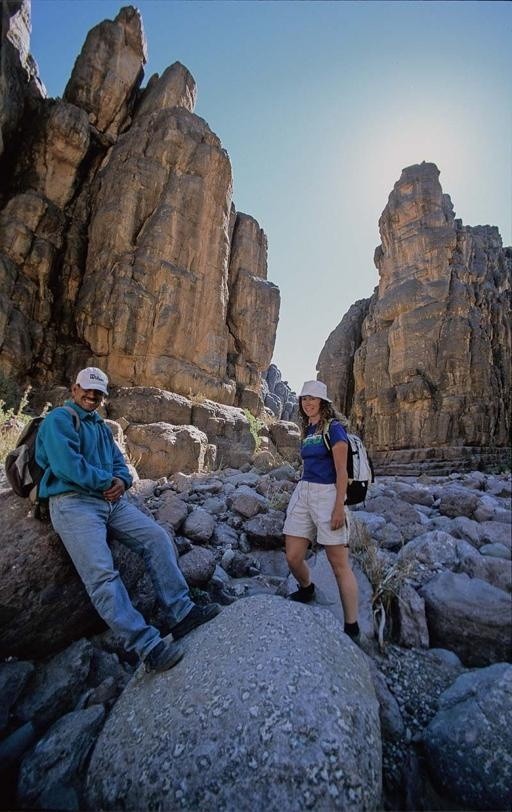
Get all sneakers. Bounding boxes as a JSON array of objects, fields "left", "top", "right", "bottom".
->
[
  {"left": 144, "top": 640, "right": 183, "bottom": 674},
  {"left": 287, "top": 584, "right": 316, "bottom": 602},
  {"left": 344, "top": 628, "right": 362, "bottom": 643},
  {"left": 172, "top": 603, "right": 222, "bottom": 641}
]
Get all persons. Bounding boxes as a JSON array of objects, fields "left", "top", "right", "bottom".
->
[
  {"left": 33, "top": 367, "right": 223, "bottom": 673},
  {"left": 283, "top": 381, "right": 361, "bottom": 645}
]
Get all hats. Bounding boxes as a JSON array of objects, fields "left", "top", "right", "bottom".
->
[
  {"left": 299, "top": 381, "right": 333, "bottom": 404},
  {"left": 76, "top": 367, "right": 109, "bottom": 396}
]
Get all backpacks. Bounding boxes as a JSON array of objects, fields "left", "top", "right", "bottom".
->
[
  {"left": 322, "top": 418, "right": 374, "bottom": 508},
  {"left": 5, "top": 406, "right": 80, "bottom": 498}
]
